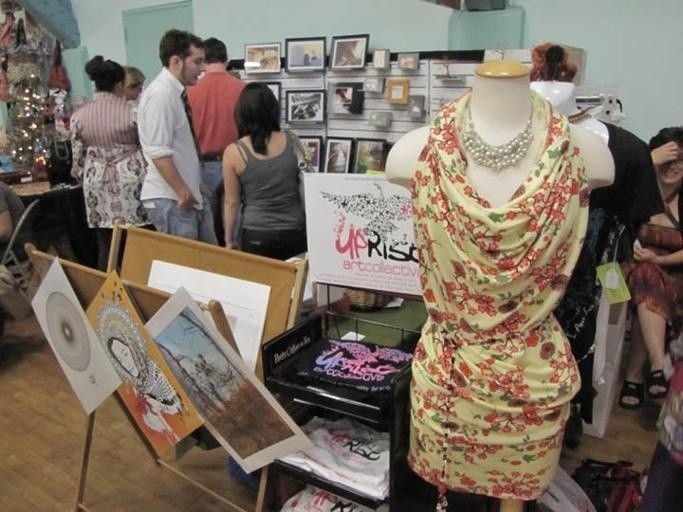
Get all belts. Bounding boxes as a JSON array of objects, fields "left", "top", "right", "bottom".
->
[{"left": 201, "top": 155, "right": 223, "bottom": 164}]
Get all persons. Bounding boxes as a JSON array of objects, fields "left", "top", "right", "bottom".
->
[
  {"left": 528, "top": 42, "right": 664, "bottom": 450},
  {"left": 220, "top": 81, "right": 311, "bottom": 260},
  {"left": 0, "top": 179, "right": 42, "bottom": 270},
  {"left": 68, "top": 56, "right": 157, "bottom": 276},
  {"left": 617, "top": 127, "right": 681, "bottom": 409},
  {"left": 136, "top": 29, "right": 220, "bottom": 247},
  {"left": 184, "top": 37, "right": 248, "bottom": 248},
  {"left": 105, "top": 335, "right": 174, "bottom": 403},
  {"left": 122, "top": 65, "right": 145, "bottom": 104},
  {"left": 385, "top": 61, "right": 616, "bottom": 502}
]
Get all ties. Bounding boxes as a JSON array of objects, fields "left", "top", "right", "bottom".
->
[{"left": 180, "top": 89, "right": 205, "bottom": 170}]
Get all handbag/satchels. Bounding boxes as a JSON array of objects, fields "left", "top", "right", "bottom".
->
[{"left": 282, "top": 128, "right": 317, "bottom": 217}]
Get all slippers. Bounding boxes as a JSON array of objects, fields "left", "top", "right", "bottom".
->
[
  {"left": 618, "top": 378, "right": 645, "bottom": 410},
  {"left": 646, "top": 370, "right": 668, "bottom": 399}
]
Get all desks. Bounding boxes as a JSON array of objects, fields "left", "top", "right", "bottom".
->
[{"left": 10, "top": 180, "right": 83, "bottom": 262}]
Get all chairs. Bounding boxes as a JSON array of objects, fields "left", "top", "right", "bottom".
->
[{"left": 0, "top": 198, "right": 41, "bottom": 305}]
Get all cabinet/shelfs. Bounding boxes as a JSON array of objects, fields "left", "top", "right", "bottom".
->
[{"left": 260, "top": 310, "right": 436, "bottom": 512}]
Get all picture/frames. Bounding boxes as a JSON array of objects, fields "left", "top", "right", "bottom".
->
[{"left": 242, "top": 31, "right": 425, "bottom": 174}]
